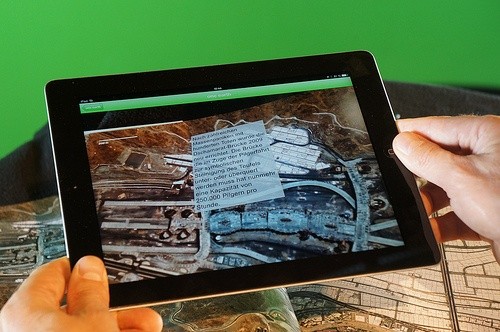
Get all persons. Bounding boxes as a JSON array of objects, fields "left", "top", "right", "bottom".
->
[{"left": 0, "top": 112, "right": 500, "bottom": 332}]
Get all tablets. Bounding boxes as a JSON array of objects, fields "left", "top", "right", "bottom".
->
[{"left": 44, "top": 50, "right": 441, "bottom": 312}]
